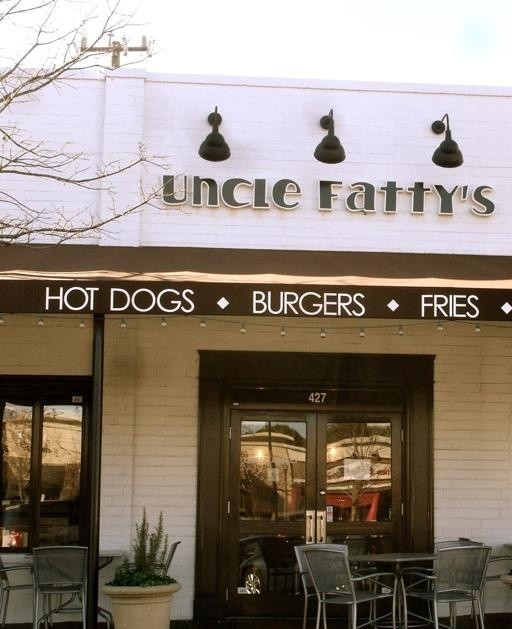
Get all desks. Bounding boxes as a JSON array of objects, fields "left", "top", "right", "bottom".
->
[{"left": 23, "top": 550, "right": 123, "bottom": 626}]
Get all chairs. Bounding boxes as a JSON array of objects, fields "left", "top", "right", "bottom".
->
[
  {"left": 293, "top": 538, "right": 490, "bottom": 629},
  {"left": 0, "top": 544, "right": 88, "bottom": 629}
]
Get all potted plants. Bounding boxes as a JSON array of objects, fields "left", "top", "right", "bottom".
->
[{"left": 97, "top": 504, "right": 183, "bottom": 629}]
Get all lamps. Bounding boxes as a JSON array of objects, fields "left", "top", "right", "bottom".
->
[
  {"left": 312, "top": 109, "right": 347, "bottom": 164},
  {"left": 430, "top": 113, "right": 465, "bottom": 168},
  {"left": 198, "top": 104, "right": 233, "bottom": 163}
]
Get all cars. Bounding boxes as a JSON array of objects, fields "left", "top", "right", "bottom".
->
[{"left": 238, "top": 535, "right": 396, "bottom": 595}]
